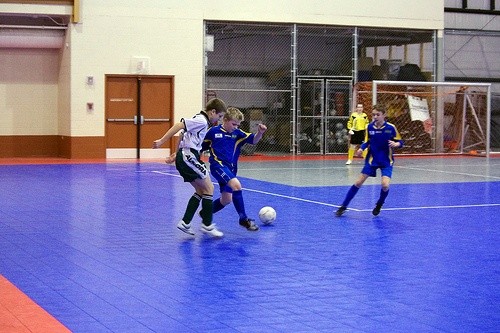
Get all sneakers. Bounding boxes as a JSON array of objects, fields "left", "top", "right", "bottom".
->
[
  {"left": 336, "top": 206, "right": 346, "bottom": 216},
  {"left": 177, "top": 219, "right": 196, "bottom": 236},
  {"left": 200, "top": 223, "right": 224, "bottom": 237},
  {"left": 346, "top": 160, "right": 352, "bottom": 164},
  {"left": 372, "top": 206, "right": 381, "bottom": 215},
  {"left": 238, "top": 219, "right": 260, "bottom": 230}
]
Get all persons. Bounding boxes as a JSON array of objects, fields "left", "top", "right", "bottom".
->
[
  {"left": 197, "top": 107, "right": 267, "bottom": 232},
  {"left": 153, "top": 98, "right": 227, "bottom": 238},
  {"left": 336, "top": 104, "right": 404, "bottom": 216},
  {"left": 345, "top": 102, "right": 370, "bottom": 164}
]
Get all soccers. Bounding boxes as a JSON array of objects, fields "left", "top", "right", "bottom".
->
[
  {"left": 258, "top": 205, "right": 276, "bottom": 225},
  {"left": 300, "top": 109, "right": 347, "bottom": 145}
]
